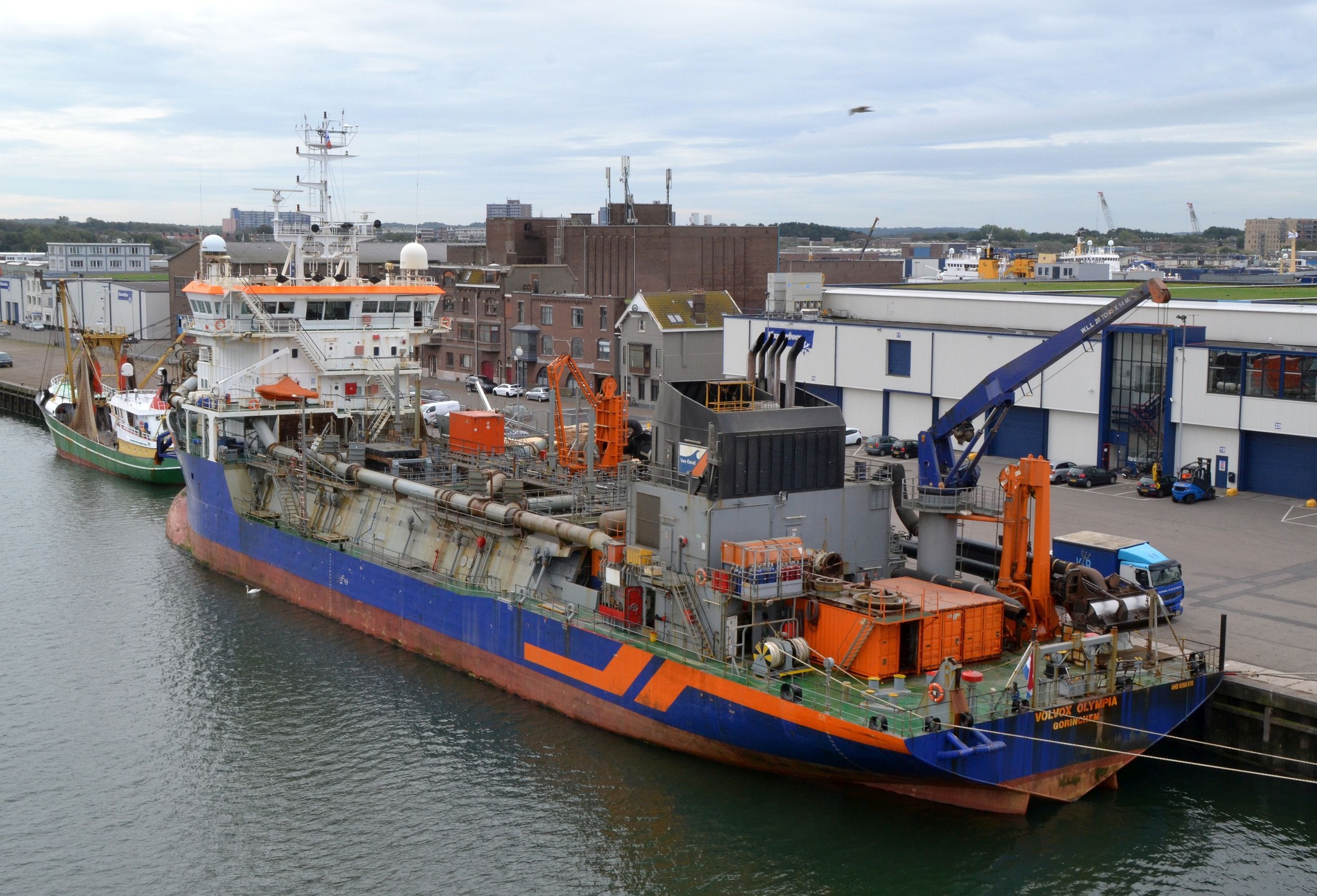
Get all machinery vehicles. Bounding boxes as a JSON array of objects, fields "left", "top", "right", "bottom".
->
[
  {"left": 858, "top": 217, "right": 880, "bottom": 260},
  {"left": 1171, "top": 457, "right": 1216, "bottom": 504}
]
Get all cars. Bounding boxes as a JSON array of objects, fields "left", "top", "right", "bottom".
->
[
  {"left": 409, "top": 386, "right": 451, "bottom": 402},
  {"left": 21, "top": 321, "right": 45, "bottom": 332},
  {"left": 0, "top": 351, "right": 13, "bottom": 367},
  {"left": 0, "top": 327, "right": 10, "bottom": 336},
  {"left": 492, "top": 382, "right": 523, "bottom": 398},
  {"left": 95, "top": 330, "right": 138, "bottom": 344},
  {"left": 502, "top": 404, "right": 533, "bottom": 438},
  {"left": 1049, "top": 459, "right": 1118, "bottom": 489},
  {"left": 1136, "top": 473, "right": 1180, "bottom": 498},
  {"left": 525, "top": 386, "right": 550, "bottom": 402},
  {"left": 430, "top": 411, "right": 449, "bottom": 428},
  {"left": 846, "top": 427, "right": 917, "bottom": 459},
  {"left": 1132, "top": 449, "right": 1163, "bottom": 463}
]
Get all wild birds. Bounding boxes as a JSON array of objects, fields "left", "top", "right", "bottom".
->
[{"left": 848, "top": 105, "right": 874, "bottom": 117}]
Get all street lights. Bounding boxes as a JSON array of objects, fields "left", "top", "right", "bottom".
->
[
  {"left": 515, "top": 345, "right": 523, "bottom": 423},
  {"left": 1174, "top": 314, "right": 1187, "bottom": 479},
  {"left": 613, "top": 332, "right": 628, "bottom": 401}
]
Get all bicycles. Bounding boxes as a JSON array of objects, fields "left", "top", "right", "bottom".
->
[{"left": 628, "top": 397, "right": 639, "bottom": 407}]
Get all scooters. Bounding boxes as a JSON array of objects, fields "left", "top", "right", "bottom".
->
[{"left": 1121, "top": 463, "right": 1147, "bottom": 481}]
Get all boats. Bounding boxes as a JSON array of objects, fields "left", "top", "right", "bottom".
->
[
  {"left": 254, "top": 376, "right": 319, "bottom": 402},
  {"left": 35, "top": 108, "right": 454, "bottom": 489},
  {"left": 164, "top": 276, "right": 1229, "bottom": 818},
  {"left": 907, "top": 230, "right": 1317, "bottom": 288}
]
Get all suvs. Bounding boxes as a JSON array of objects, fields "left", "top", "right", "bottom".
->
[{"left": 465, "top": 375, "right": 499, "bottom": 394}]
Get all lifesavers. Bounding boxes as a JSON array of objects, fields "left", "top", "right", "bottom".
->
[
  {"left": 215, "top": 320, "right": 226, "bottom": 330},
  {"left": 928, "top": 682, "right": 944, "bottom": 702},
  {"left": 442, "top": 317, "right": 450, "bottom": 327},
  {"left": 248, "top": 400, "right": 258, "bottom": 409},
  {"left": 695, "top": 568, "right": 706, "bottom": 586}
]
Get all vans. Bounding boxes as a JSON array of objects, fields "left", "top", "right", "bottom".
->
[{"left": 421, "top": 400, "right": 460, "bottom": 425}]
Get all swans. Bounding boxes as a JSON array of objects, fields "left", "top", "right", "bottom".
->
[{"left": 245, "top": 585, "right": 261, "bottom": 594}]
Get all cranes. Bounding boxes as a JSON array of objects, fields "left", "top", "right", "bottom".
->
[
  {"left": 1187, "top": 202, "right": 1202, "bottom": 236},
  {"left": 1097, "top": 191, "right": 1115, "bottom": 231}
]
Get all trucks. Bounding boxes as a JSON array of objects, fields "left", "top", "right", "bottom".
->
[{"left": 1052, "top": 530, "right": 1185, "bottom": 624}]
